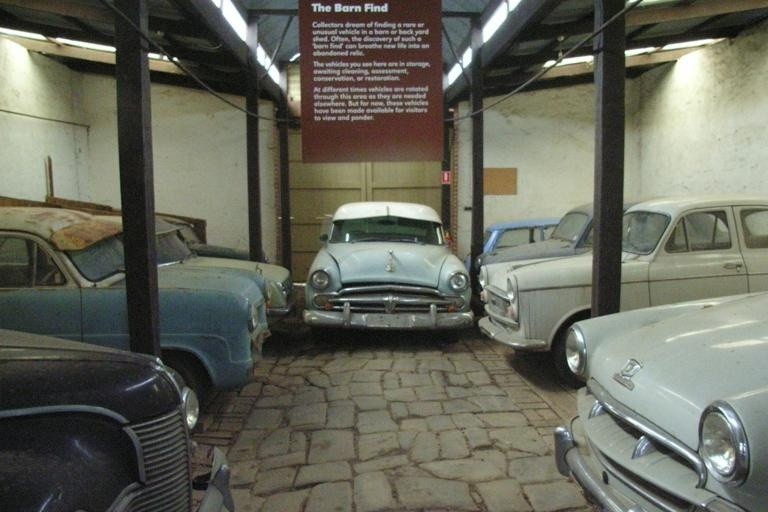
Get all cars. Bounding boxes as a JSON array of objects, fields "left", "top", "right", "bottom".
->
[
  {"left": 554, "top": 293, "right": 768, "bottom": 512},
  {"left": 0, "top": 329, "right": 235, "bottom": 512},
  {"left": 302, "top": 202, "right": 475, "bottom": 330}
]
